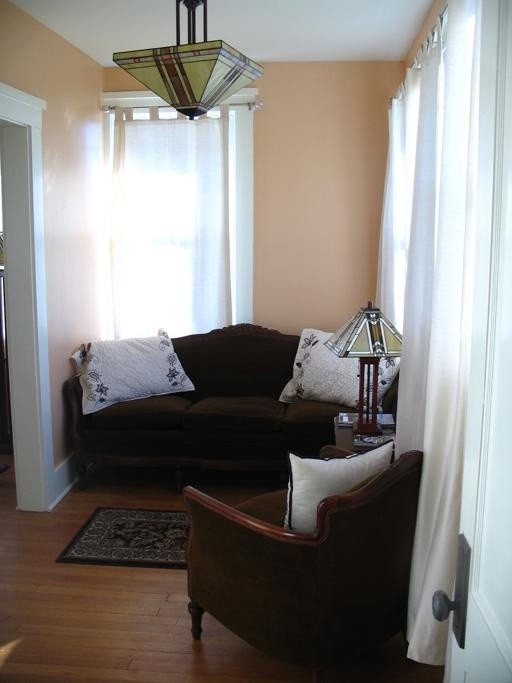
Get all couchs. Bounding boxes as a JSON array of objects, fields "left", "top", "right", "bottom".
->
[{"left": 62, "top": 323, "right": 399, "bottom": 488}]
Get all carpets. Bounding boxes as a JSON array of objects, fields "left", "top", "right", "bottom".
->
[{"left": 55, "top": 506, "right": 191, "bottom": 569}]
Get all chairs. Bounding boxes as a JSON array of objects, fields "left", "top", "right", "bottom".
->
[{"left": 184, "top": 444, "right": 423, "bottom": 683}]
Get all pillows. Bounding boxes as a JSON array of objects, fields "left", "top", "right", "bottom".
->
[
  {"left": 70, "top": 327, "right": 195, "bottom": 415},
  {"left": 283, "top": 438, "right": 395, "bottom": 534},
  {"left": 279, "top": 328, "right": 401, "bottom": 414}
]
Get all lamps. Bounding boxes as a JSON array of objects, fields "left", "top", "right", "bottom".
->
[
  {"left": 112, "top": 0, "right": 263, "bottom": 120},
  {"left": 322, "top": 300, "right": 402, "bottom": 436}
]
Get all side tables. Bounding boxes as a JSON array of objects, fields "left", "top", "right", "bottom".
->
[{"left": 333, "top": 415, "right": 395, "bottom": 450}]
